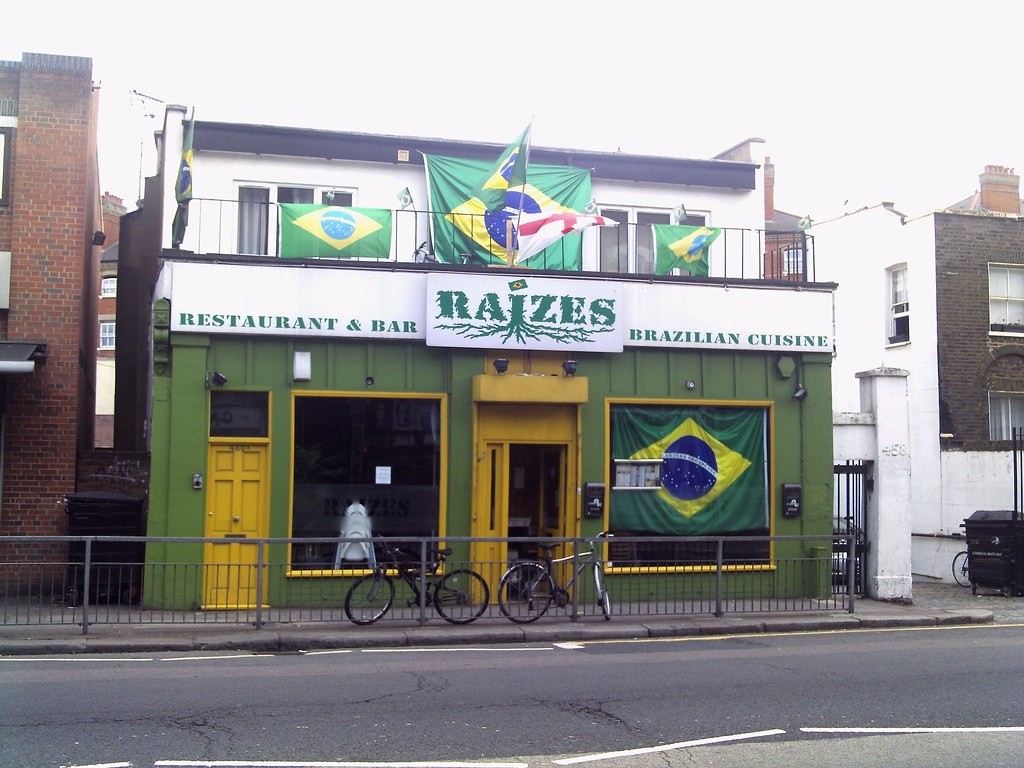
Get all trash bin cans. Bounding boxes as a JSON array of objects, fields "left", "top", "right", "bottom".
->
[
  {"left": 959, "top": 509, "right": 1024, "bottom": 597},
  {"left": 64, "top": 491, "right": 145, "bottom": 605}
]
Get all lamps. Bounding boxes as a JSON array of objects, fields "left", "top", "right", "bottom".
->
[
  {"left": 793, "top": 384, "right": 808, "bottom": 402},
  {"left": 492, "top": 358, "right": 509, "bottom": 376},
  {"left": 561, "top": 360, "right": 578, "bottom": 377},
  {"left": 88, "top": 230, "right": 106, "bottom": 247},
  {"left": 204, "top": 370, "right": 227, "bottom": 389},
  {"left": 940, "top": 423, "right": 955, "bottom": 438}
]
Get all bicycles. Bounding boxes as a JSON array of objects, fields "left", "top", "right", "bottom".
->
[
  {"left": 343, "top": 532, "right": 489, "bottom": 625},
  {"left": 953, "top": 551, "right": 972, "bottom": 588},
  {"left": 496, "top": 531, "right": 617, "bottom": 620}
]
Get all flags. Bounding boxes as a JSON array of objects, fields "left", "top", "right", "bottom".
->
[
  {"left": 582, "top": 199, "right": 598, "bottom": 214},
  {"left": 651, "top": 224, "right": 721, "bottom": 276},
  {"left": 470, "top": 122, "right": 533, "bottom": 213},
  {"left": 510, "top": 212, "right": 620, "bottom": 263},
  {"left": 171, "top": 118, "right": 194, "bottom": 245},
  {"left": 326, "top": 188, "right": 335, "bottom": 200},
  {"left": 796, "top": 216, "right": 811, "bottom": 231},
  {"left": 422, "top": 153, "right": 591, "bottom": 271},
  {"left": 673, "top": 205, "right": 687, "bottom": 223},
  {"left": 610, "top": 407, "right": 769, "bottom": 536},
  {"left": 278, "top": 203, "right": 392, "bottom": 258},
  {"left": 396, "top": 188, "right": 412, "bottom": 210}
]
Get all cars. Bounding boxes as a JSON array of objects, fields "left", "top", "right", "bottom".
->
[{"left": 832, "top": 517, "right": 864, "bottom": 578}]
